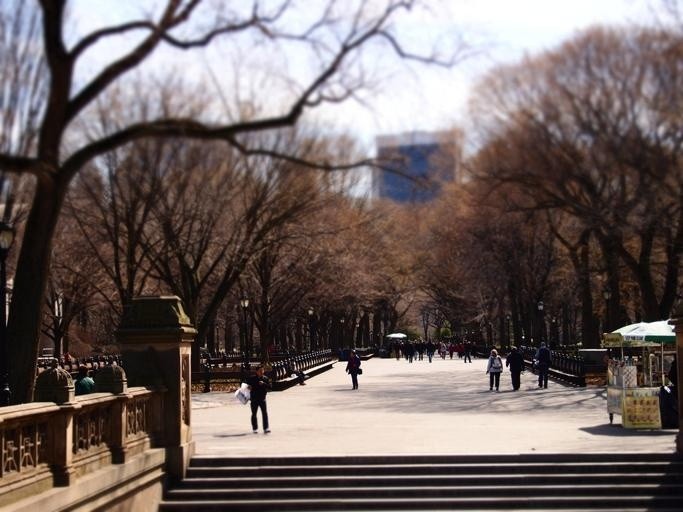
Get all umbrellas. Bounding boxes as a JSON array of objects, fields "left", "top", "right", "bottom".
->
[{"left": 612, "top": 322, "right": 677, "bottom": 386}]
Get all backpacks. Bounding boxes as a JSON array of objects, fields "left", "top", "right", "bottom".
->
[{"left": 492, "top": 356, "right": 502, "bottom": 369}]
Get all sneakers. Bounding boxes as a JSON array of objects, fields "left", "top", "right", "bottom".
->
[
  {"left": 253, "top": 429, "right": 259, "bottom": 432},
  {"left": 264, "top": 428, "right": 271, "bottom": 433},
  {"left": 351, "top": 386, "right": 359, "bottom": 390},
  {"left": 490, "top": 388, "right": 499, "bottom": 391}
]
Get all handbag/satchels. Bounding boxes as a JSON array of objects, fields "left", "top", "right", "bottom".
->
[{"left": 357, "top": 368, "right": 362, "bottom": 374}]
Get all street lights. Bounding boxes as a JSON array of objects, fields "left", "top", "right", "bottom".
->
[
  {"left": 240, "top": 295, "right": 250, "bottom": 369},
  {"left": 356, "top": 324, "right": 360, "bottom": 348},
  {"left": 537, "top": 300, "right": 545, "bottom": 343},
  {"left": 505, "top": 314, "right": 511, "bottom": 346},
  {"left": 488, "top": 321, "right": 493, "bottom": 346},
  {"left": 307, "top": 307, "right": 315, "bottom": 352},
  {"left": 339, "top": 315, "right": 345, "bottom": 348},
  {"left": 601, "top": 286, "right": 611, "bottom": 331}
]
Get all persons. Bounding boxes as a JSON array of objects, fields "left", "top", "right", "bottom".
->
[
  {"left": 346, "top": 352, "right": 360, "bottom": 390},
  {"left": 390, "top": 339, "right": 510, "bottom": 362},
  {"left": 286, "top": 359, "right": 309, "bottom": 385},
  {"left": 486, "top": 349, "right": 503, "bottom": 391},
  {"left": 66, "top": 352, "right": 72, "bottom": 371},
  {"left": 75, "top": 366, "right": 93, "bottom": 395},
  {"left": 535, "top": 342, "right": 553, "bottom": 389},
  {"left": 247, "top": 366, "right": 272, "bottom": 434},
  {"left": 506, "top": 346, "right": 525, "bottom": 390}
]
